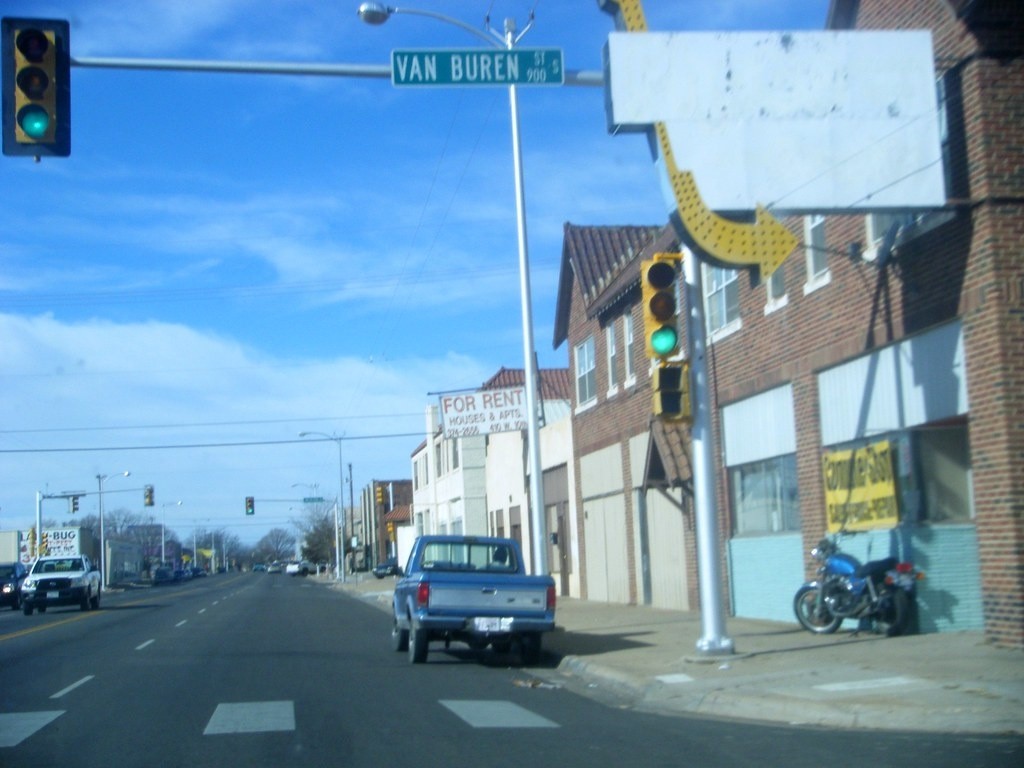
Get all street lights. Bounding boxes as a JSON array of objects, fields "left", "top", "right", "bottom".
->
[
  {"left": 97, "top": 469, "right": 130, "bottom": 592},
  {"left": 299, "top": 431, "right": 346, "bottom": 584},
  {"left": 293, "top": 481, "right": 321, "bottom": 578},
  {"left": 360, "top": 0, "right": 552, "bottom": 578},
  {"left": 160, "top": 499, "right": 185, "bottom": 565}
]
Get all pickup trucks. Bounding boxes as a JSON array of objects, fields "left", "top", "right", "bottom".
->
[{"left": 386, "top": 533, "right": 555, "bottom": 665}]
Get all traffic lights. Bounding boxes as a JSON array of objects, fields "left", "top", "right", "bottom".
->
[
  {"left": 245, "top": 497, "right": 259, "bottom": 514},
  {"left": 639, "top": 257, "right": 682, "bottom": 362},
  {"left": 69, "top": 496, "right": 79, "bottom": 512},
  {"left": 376, "top": 487, "right": 383, "bottom": 504},
  {"left": 387, "top": 521, "right": 396, "bottom": 534},
  {"left": 0, "top": 17, "right": 74, "bottom": 160}
]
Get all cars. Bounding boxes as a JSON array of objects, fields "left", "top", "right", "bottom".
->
[
  {"left": 150, "top": 562, "right": 226, "bottom": 587},
  {"left": 320, "top": 558, "right": 335, "bottom": 573},
  {"left": 372, "top": 557, "right": 397, "bottom": 580},
  {"left": 287, "top": 559, "right": 318, "bottom": 576},
  {"left": 252, "top": 562, "right": 267, "bottom": 574},
  {"left": 268, "top": 562, "right": 281, "bottom": 574},
  {"left": 0, "top": 561, "right": 28, "bottom": 610},
  {"left": 20, "top": 553, "right": 101, "bottom": 616}
]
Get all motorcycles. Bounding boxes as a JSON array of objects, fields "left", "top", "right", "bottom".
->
[{"left": 792, "top": 522, "right": 923, "bottom": 640}]
